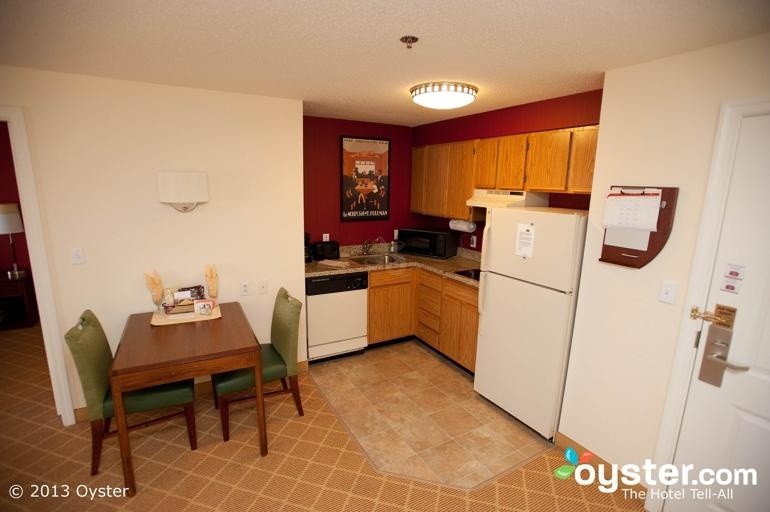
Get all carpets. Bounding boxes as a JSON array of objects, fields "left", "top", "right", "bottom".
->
[{"left": 309, "top": 337, "right": 555, "bottom": 493}]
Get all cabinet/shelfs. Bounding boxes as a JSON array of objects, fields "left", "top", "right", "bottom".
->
[
  {"left": 369, "top": 267, "right": 414, "bottom": 344},
  {"left": 425, "top": 139, "right": 473, "bottom": 221},
  {"left": 410, "top": 145, "right": 428, "bottom": 214},
  {"left": 475, "top": 136, "right": 528, "bottom": 190},
  {"left": 413, "top": 267, "right": 442, "bottom": 350},
  {"left": 442, "top": 281, "right": 479, "bottom": 373},
  {"left": 527, "top": 131, "right": 597, "bottom": 191}
]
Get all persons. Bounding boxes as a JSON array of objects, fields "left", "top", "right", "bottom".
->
[
  {"left": 349, "top": 169, "right": 359, "bottom": 183},
  {"left": 375, "top": 169, "right": 384, "bottom": 185}
]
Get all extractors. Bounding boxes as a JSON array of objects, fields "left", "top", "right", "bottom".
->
[{"left": 465, "top": 189, "right": 549, "bottom": 208}]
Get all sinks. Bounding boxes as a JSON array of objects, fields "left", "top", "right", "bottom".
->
[{"left": 352, "top": 254, "right": 404, "bottom": 266}]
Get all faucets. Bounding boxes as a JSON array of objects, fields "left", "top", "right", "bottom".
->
[{"left": 362, "top": 240, "right": 370, "bottom": 254}]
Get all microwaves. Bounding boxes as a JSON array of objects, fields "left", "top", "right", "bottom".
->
[{"left": 397, "top": 229, "right": 457, "bottom": 259}]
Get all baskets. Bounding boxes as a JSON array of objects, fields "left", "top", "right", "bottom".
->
[{"left": 162, "top": 294, "right": 207, "bottom": 315}]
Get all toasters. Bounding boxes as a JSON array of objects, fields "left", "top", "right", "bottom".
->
[{"left": 312, "top": 240, "right": 340, "bottom": 260}]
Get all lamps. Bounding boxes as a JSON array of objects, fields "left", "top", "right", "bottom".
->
[
  {"left": 0, "top": 203, "right": 25, "bottom": 272},
  {"left": 408, "top": 81, "right": 480, "bottom": 109}
]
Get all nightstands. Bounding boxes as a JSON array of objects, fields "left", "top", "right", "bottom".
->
[{"left": 0, "top": 271, "right": 36, "bottom": 329}]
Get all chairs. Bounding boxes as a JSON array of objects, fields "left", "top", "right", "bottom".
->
[
  {"left": 211, "top": 286, "right": 302, "bottom": 441},
  {"left": 65, "top": 309, "right": 197, "bottom": 476}
]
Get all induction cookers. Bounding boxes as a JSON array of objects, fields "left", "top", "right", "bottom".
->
[{"left": 453, "top": 269, "right": 481, "bottom": 281}]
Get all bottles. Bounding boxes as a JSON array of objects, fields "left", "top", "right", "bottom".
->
[{"left": 199, "top": 304, "right": 212, "bottom": 316}]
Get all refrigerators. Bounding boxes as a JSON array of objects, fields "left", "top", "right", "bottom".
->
[{"left": 474, "top": 206, "right": 589, "bottom": 443}]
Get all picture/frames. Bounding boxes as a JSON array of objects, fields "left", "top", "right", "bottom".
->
[{"left": 339, "top": 134, "right": 390, "bottom": 220}]
